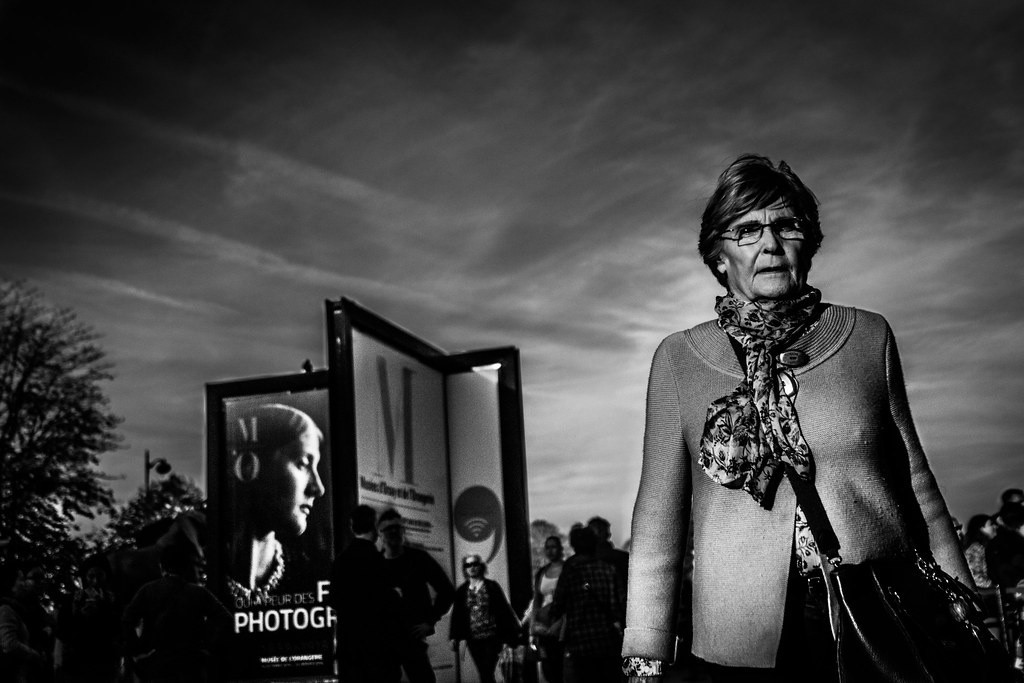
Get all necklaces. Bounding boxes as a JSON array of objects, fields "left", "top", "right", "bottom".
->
[{"left": 227, "top": 537, "right": 287, "bottom": 607}]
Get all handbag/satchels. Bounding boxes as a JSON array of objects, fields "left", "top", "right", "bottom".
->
[
  {"left": 494, "top": 644, "right": 522, "bottom": 683},
  {"left": 829, "top": 555, "right": 1015, "bottom": 682}
]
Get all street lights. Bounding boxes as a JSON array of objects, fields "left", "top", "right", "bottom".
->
[{"left": 143, "top": 448, "right": 171, "bottom": 493}]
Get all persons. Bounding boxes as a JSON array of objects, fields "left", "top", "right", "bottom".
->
[
  {"left": 332, "top": 504, "right": 457, "bottom": 683},
  {"left": 447, "top": 556, "right": 525, "bottom": 683},
  {"left": 528, "top": 517, "right": 629, "bottom": 683},
  {"left": 621, "top": 149, "right": 1024, "bottom": 683},
  {"left": 950, "top": 488, "right": 1024, "bottom": 674},
  {"left": 222, "top": 402, "right": 327, "bottom": 621},
  {"left": 0, "top": 520, "right": 236, "bottom": 683}
]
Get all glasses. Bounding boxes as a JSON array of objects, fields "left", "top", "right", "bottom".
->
[
  {"left": 464, "top": 562, "right": 478, "bottom": 568},
  {"left": 720, "top": 218, "right": 806, "bottom": 246}
]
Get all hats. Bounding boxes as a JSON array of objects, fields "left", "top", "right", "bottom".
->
[{"left": 379, "top": 509, "right": 403, "bottom": 531}]
